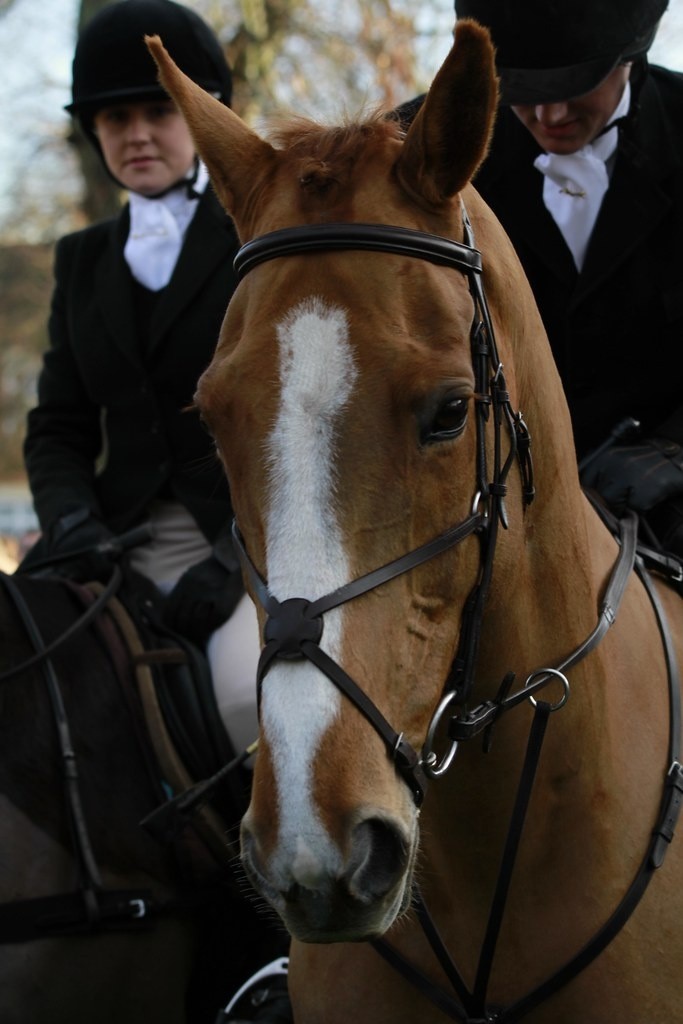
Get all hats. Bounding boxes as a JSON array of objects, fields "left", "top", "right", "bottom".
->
[{"left": 451, "top": 0, "right": 671, "bottom": 105}]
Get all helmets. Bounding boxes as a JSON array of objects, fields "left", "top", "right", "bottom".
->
[{"left": 64, "top": 0, "right": 234, "bottom": 110}]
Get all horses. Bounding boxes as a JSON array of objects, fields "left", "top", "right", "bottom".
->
[
  {"left": 142, "top": 19, "right": 683, "bottom": 1024},
  {"left": 0, "top": 569, "right": 295, "bottom": 1024}
]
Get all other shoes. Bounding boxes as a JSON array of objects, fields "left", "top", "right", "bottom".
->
[{"left": 220, "top": 935, "right": 293, "bottom": 1024}]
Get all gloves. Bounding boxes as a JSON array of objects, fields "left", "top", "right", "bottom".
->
[
  {"left": 582, "top": 436, "right": 683, "bottom": 520},
  {"left": 53, "top": 513, "right": 123, "bottom": 578}
]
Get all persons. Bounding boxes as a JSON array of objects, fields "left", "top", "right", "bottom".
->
[
  {"left": 388, "top": 0, "right": 683, "bottom": 597},
  {"left": 22, "top": 1, "right": 262, "bottom": 770}
]
